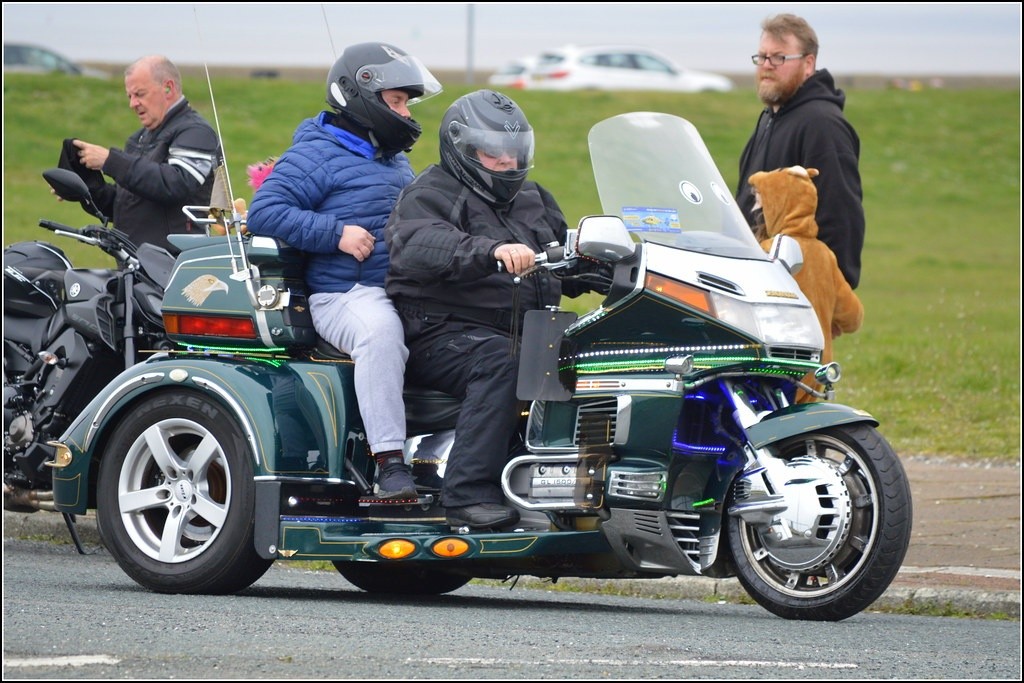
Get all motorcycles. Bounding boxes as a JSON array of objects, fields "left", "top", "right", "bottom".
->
[
  {"left": 51, "top": 110, "right": 913, "bottom": 623},
  {"left": 5, "top": 167, "right": 178, "bottom": 555}
]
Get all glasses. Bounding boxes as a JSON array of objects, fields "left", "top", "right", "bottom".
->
[
  {"left": 475, "top": 143, "right": 522, "bottom": 159},
  {"left": 751, "top": 54, "right": 802, "bottom": 66}
]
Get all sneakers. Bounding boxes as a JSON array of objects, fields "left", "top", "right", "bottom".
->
[
  {"left": 373, "top": 463, "right": 419, "bottom": 501},
  {"left": 446, "top": 503, "right": 520, "bottom": 529},
  {"left": 412, "top": 462, "right": 444, "bottom": 493}
]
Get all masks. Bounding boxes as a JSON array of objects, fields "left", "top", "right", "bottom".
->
[{"left": 750, "top": 206, "right": 765, "bottom": 227}]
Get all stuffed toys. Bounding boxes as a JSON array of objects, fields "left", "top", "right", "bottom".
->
[{"left": 747, "top": 164, "right": 863, "bottom": 404}]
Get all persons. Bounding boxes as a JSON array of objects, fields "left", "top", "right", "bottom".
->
[
  {"left": 49, "top": 55, "right": 221, "bottom": 273},
  {"left": 736, "top": 15, "right": 866, "bottom": 290},
  {"left": 246, "top": 42, "right": 442, "bottom": 502},
  {"left": 384, "top": 89, "right": 570, "bottom": 528}
]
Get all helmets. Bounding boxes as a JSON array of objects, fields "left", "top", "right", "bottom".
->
[
  {"left": 4, "top": 239, "right": 74, "bottom": 319},
  {"left": 439, "top": 88, "right": 536, "bottom": 208},
  {"left": 325, "top": 42, "right": 444, "bottom": 153}
]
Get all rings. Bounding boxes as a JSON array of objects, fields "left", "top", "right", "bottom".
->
[{"left": 510, "top": 250, "right": 517, "bottom": 255}]
